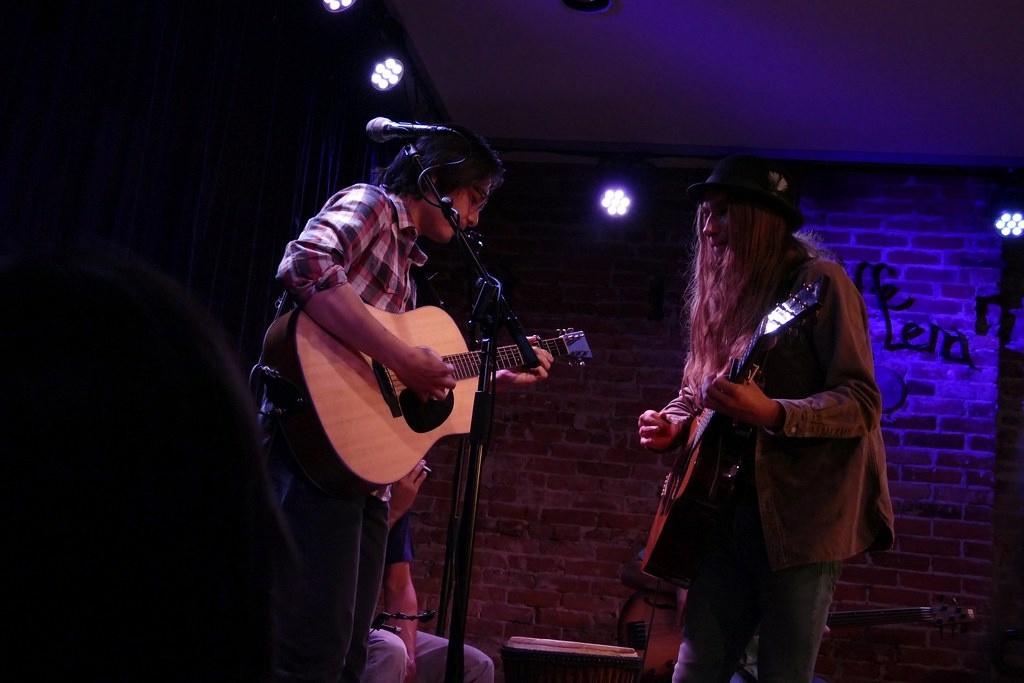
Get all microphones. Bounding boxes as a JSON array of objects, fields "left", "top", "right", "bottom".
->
[
  {"left": 419, "top": 465, "right": 432, "bottom": 480},
  {"left": 365, "top": 117, "right": 453, "bottom": 142}
]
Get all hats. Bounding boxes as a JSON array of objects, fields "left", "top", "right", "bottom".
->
[{"left": 686, "top": 153, "right": 806, "bottom": 233}]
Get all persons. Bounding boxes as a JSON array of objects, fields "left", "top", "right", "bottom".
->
[
  {"left": 621, "top": 544, "right": 829, "bottom": 683},
  {"left": 357, "top": 457, "right": 497, "bottom": 683},
  {"left": 249, "top": 122, "right": 555, "bottom": 682},
  {"left": 638, "top": 158, "right": 895, "bottom": 683}
]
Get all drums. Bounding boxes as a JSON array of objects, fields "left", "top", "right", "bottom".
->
[{"left": 500, "top": 636, "right": 641, "bottom": 683}]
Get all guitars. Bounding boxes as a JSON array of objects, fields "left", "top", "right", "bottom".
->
[
  {"left": 617, "top": 587, "right": 975, "bottom": 683},
  {"left": 260, "top": 297, "right": 593, "bottom": 499},
  {"left": 640, "top": 280, "right": 826, "bottom": 591}
]
